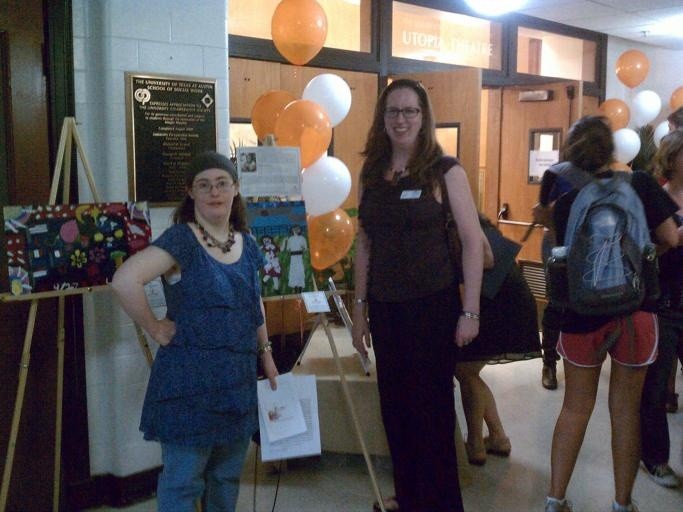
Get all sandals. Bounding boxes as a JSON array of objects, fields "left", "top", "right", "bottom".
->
[{"left": 464, "top": 437, "right": 511, "bottom": 465}]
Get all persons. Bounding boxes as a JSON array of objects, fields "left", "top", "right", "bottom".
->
[
  {"left": 110, "top": 153, "right": 279, "bottom": 512},
  {"left": 538, "top": 108, "right": 683, "bottom": 511},
  {"left": 352, "top": 79, "right": 484, "bottom": 511},
  {"left": 446, "top": 205, "right": 541, "bottom": 466}
]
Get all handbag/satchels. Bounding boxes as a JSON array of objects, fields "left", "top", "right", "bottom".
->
[{"left": 436, "top": 156, "right": 495, "bottom": 272}]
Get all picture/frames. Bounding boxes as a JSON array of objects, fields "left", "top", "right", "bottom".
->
[{"left": 125, "top": 71, "right": 218, "bottom": 207}]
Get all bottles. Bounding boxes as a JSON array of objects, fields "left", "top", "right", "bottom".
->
[{"left": 547, "top": 246, "right": 569, "bottom": 299}]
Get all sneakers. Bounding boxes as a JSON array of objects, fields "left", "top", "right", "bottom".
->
[{"left": 638, "top": 459, "right": 677, "bottom": 487}]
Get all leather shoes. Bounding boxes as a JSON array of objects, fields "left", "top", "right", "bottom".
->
[{"left": 542, "top": 363, "right": 556, "bottom": 390}]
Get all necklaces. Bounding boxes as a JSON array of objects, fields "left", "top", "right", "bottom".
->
[
  {"left": 387, "top": 163, "right": 412, "bottom": 187},
  {"left": 191, "top": 217, "right": 236, "bottom": 254}
]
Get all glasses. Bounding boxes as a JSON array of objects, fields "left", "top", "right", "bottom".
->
[
  {"left": 380, "top": 105, "right": 421, "bottom": 120},
  {"left": 189, "top": 180, "right": 235, "bottom": 194}
]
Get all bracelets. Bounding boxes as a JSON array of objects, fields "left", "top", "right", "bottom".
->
[
  {"left": 256, "top": 340, "right": 274, "bottom": 354},
  {"left": 352, "top": 296, "right": 367, "bottom": 306},
  {"left": 461, "top": 310, "right": 481, "bottom": 321}
]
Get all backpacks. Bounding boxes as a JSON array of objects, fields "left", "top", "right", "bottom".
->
[{"left": 538, "top": 161, "right": 663, "bottom": 317}]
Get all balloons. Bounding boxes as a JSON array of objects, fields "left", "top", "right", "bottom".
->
[
  {"left": 249, "top": 1, "right": 355, "bottom": 273},
  {"left": 596, "top": 48, "right": 683, "bottom": 173}
]
